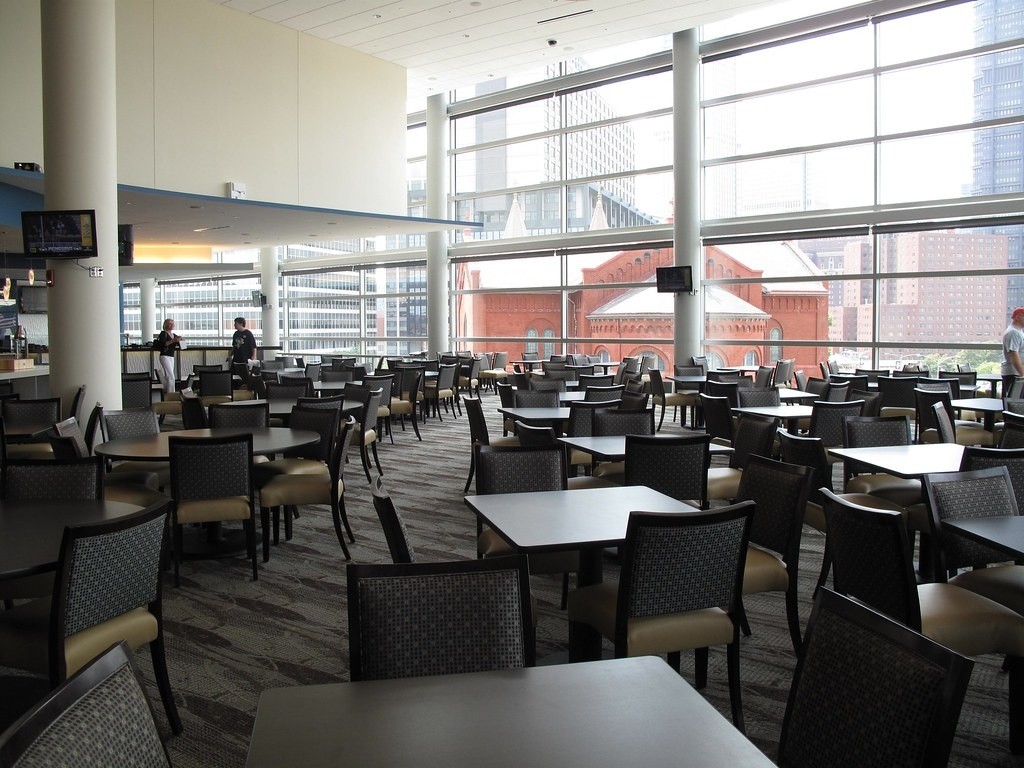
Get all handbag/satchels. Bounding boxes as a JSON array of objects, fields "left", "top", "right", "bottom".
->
[{"left": 152, "top": 331, "right": 170, "bottom": 353}]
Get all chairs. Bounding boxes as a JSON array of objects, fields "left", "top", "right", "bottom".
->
[{"left": 0, "top": 347, "right": 1024, "bottom": 768}]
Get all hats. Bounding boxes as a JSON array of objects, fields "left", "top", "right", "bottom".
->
[{"left": 1011, "top": 307, "right": 1024, "bottom": 318}]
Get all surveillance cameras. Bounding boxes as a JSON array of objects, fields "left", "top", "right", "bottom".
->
[{"left": 548, "top": 40, "right": 558, "bottom": 47}]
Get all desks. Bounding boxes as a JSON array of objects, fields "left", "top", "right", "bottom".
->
[
  {"left": 311, "top": 381, "right": 362, "bottom": 396},
  {"left": 950, "top": 397, "right": 1004, "bottom": 447},
  {"left": 565, "top": 381, "right": 578, "bottom": 387},
  {"left": 220, "top": 399, "right": 365, "bottom": 460},
  {"left": 0, "top": 365, "right": 50, "bottom": 381},
  {"left": 497, "top": 407, "right": 570, "bottom": 437},
  {"left": 976, "top": 373, "right": 1003, "bottom": 398},
  {"left": 508, "top": 360, "right": 550, "bottom": 377},
  {"left": 666, "top": 375, "right": 707, "bottom": 430},
  {"left": 590, "top": 361, "right": 621, "bottom": 375},
  {"left": 779, "top": 388, "right": 820, "bottom": 406},
  {"left": 1, "top": 500, "right": 148, "bottom": 614},
  {"left": 319, "top": 362, "right": 333, "bottom": 367},
  {"left": 94, "top": 427, "right": 321, "bottom": 561},
  {"left": 262, "top": 367, "right": 305, "bottom": 384},
  {"left": 941, "top": 514, "right": 1024, "bottom": 768},
  {"left": 461, "top": 477, "right": 703, "bottom": 662},
  {"left": 828, "top": 442, "right": 965, "bottom": 584},
  {"left": 556, "top": 436, "right": 736, "bottom": 463},
  {"left": 240, "top": 655, "right": 779, "bottom": 768},
  {"left": 717, "top": 366, "right": 776, "bottom": 376},
  {"left": 438, "top": 364, "right": 446, "bottom": 369},
  {"left": 731, "top": 405, "right": 814, "bottom": 436},
  {"left": 558, "top": 391, "right": 586, "bottom": 408},
  {"left": 425, "top": 370, "right": 439, "bottom": 377}
]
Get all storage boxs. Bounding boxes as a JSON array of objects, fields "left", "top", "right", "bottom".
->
[{"left": 0, "top": 359, "right": 34, "bottom": 370}]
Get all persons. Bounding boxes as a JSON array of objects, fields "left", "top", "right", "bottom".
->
[
  {"left": 1001, "top": 308, "right": 1024, "bottom": 398},
  {"left": 159, "top": 319, "right": 183, "bottom": 400},
  {"left": 31, "top": 217, "right": 68, "bottom": 245},
  {"left": 225, "top": 318, "right": 257, "bottom": 377}
]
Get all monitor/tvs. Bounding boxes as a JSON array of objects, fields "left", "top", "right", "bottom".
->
[
  {"left": 118, "top": 224, "right": 134, "bottom": 266},
  {"left": 656, "top": 265, "right": 692, "bottom": 294},
  {"left": 22, "top": 209, "right": 98, "bottom": 259}
]
